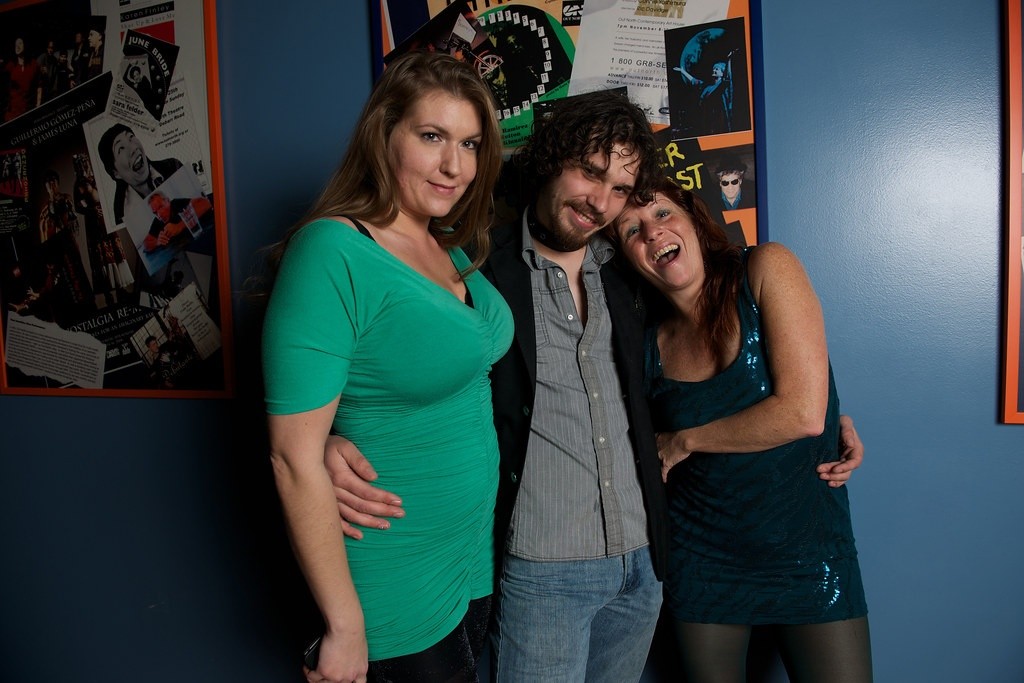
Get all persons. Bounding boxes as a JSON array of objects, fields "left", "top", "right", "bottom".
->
[
  {"left": 96, "top": 123, "right": 184, "bottom": 224},
  {"left": 122, "top": 58, "right": 160, "bottom": 121},
  {"left": 149, "top": 256, "right": 187, "bottom": 299},
  {"left": 709, "top": 152, "right": 756, "bottom": 210},
  {"left": 0, "top": 25, "right": 104, "bottom": 125},
  {"left": 1, "top": 150, "right": 144, "bottom": 320},
  {"left": 145, "top": 188, "right": 216, "bottom": 254},
  {"left": 144, "top": 326, "right": 193, "bottom": 391},
  {"left": 263, "top": 45, "right": 875, "bottom": 683},
  {"left": 673, "top": 52, "right": 743, "bottom": 134}
]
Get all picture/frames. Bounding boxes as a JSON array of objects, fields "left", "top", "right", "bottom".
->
[
  {"left": 999, "top": 0, "right": 1024, "bottom": 425},
  {"left": 0, "top": 0, "right": 236, "bottom": 399},
  {"left": 367, "top": 0, "right": 768, "bottom": 248}
]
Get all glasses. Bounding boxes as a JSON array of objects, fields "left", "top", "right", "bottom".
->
[{"left": 720, "top": 178, "right": 740, "bottom": 186}]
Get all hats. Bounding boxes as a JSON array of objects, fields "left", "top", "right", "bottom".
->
[
  {"left": 713, "top": 148, "right": 748, "bottom": 174},
  {"left": 713, "top": 62, "right": 726, "bottom": 72}
]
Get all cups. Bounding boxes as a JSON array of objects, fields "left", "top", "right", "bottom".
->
[{"left": 178, "top": 203, "right": 202, "bottom": 237}]
